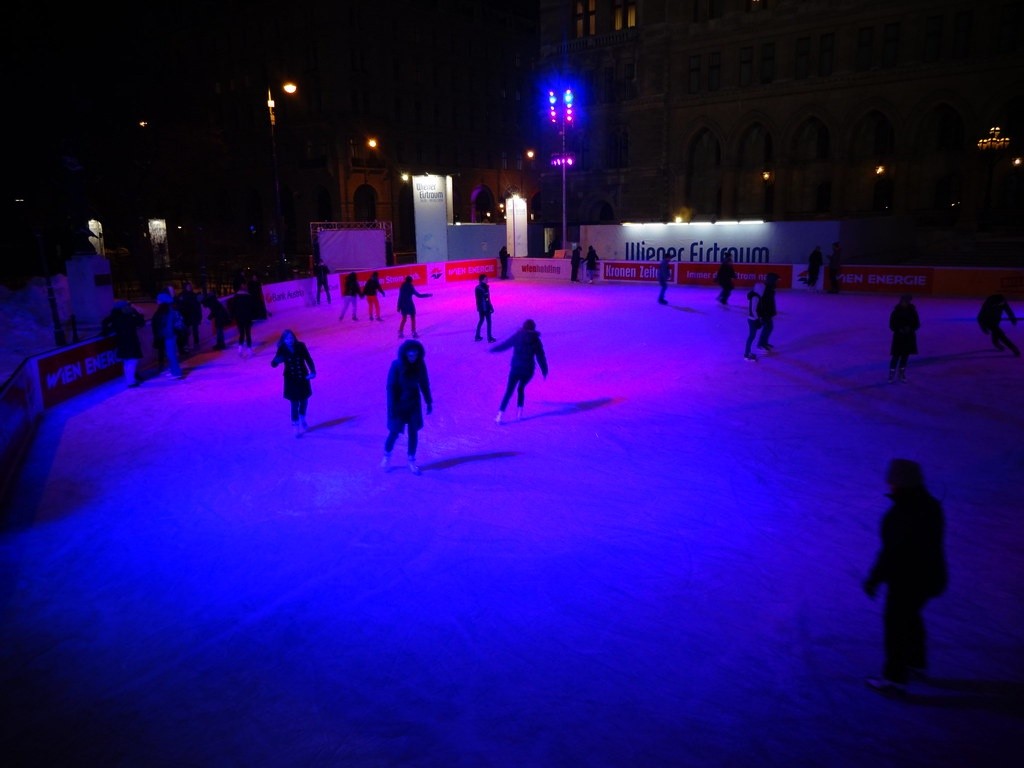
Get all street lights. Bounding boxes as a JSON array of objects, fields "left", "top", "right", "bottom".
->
[
  {"left": 549, "top": 89, "right": 575, "bottom": 250},
  {"left": 391, "top": 169, "right": 410, "bottom": 252},
  {"left": 267, "top": 83, "right": 297, "bottom": 283},
  {"left": 520, "top": 151, "right": 534, "bottom": 197}
]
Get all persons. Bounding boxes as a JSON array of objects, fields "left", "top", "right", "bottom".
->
[
  {"left": 271, "top": 329, "right": 316, "bottom": 423},
  {"left": 570, "top": 246, "right": 600, "bottom": 286},
  {"left": 805, "top": 242, "right": 843, "bottom": 295},
  {"left": 486, "top": 320, "right": 549, "bottom": 421},
  {"left": 889, "top": 294, "right": 919, "bottom": 383},
  {"left": 235, "top": 270, "right": 266, "bottom": 354},
  {"left": 974, "top": 285, "right": 1018, "bottom": 358},
  {"left": 154, "top": 286, "right": 186, "bottom": 379},
  {"left": 379, "top": 338, "right": 433, "bottom": 474},
  {"left": 858, "top": 457, "right": 955, "bottom": 701},
  {"left": 742, "top": 274, "right": 777, "bottom": 356},
  {"left": 314, "top": 259, "right": 331, "bottom": 304},
  {"left": 338, "top": 272, "right": 385, "bottom": 322},
  {"left": 499, "top": 246, "right": 511, "bottom": 279},
  {"left": 657, "top": 254, "right": 673, "bottom": 304},
  {"left": 715, "top": 253, "right": 739, "bottom": 305},
  {"left": 197, "top": 290, "right": 228, "bottom": 350},
  {"left": 184, "top": 280, "right": 202, "bottom": 352},
  {"left": 102, "top": 300, "right": 146, "bottom": 387},
  {"left": 396, "top": 275, "right": 432, "bottom": 338},
  {"left": 474, "top": 273, "right": 496, "bottom": 343}
]
